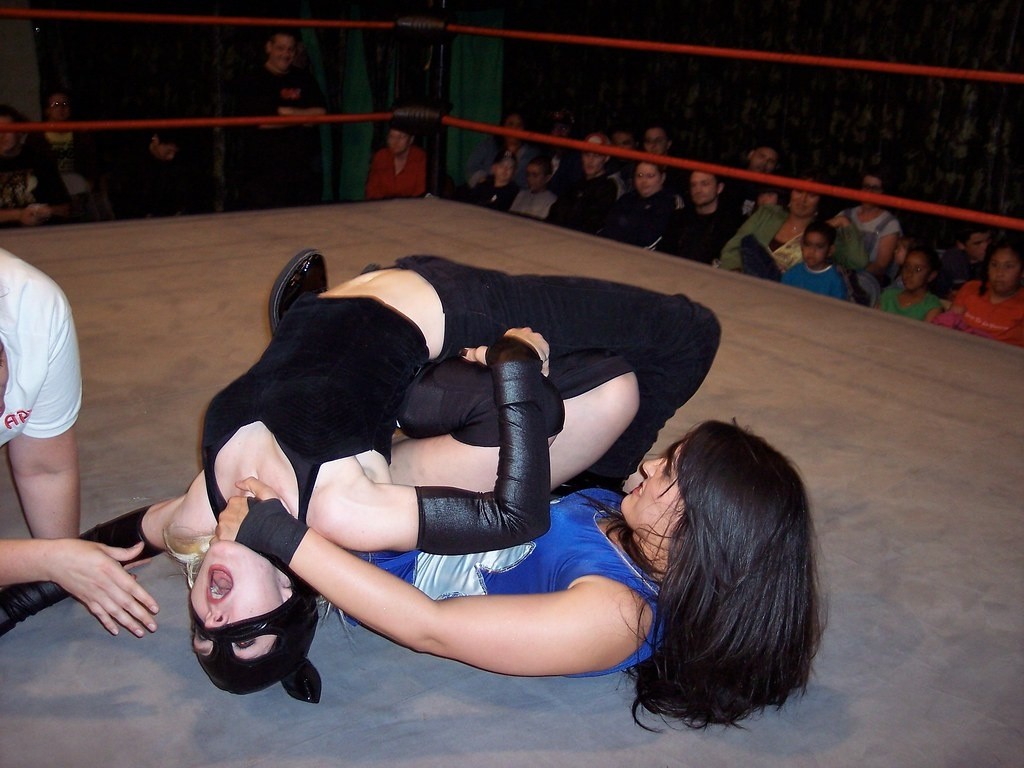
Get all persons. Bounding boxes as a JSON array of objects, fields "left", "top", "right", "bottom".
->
[
  {"left": 0, "top": 255, "right": 723, "bottom": 703},
  {"left": 363, "top": 114, "right": 428, "bottom": 203},
  {"left": 209, "top": 247, "right": 831, "bottom": 736},
  {"left": 0, "top": 23, "right": 329, "bottom": 227},
  {"left": 452, "top": 113, "right": 1024, "bottom": 345},
  {"left": 0, "top": 245, "right": 160, "bottom": 638}
]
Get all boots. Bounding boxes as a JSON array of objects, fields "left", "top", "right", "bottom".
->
[
  {"left": 545, "top": 346, "right": 635, "bottom": 400},
  {"left": 267, "top": 247, "right": 564, "bottom": 447}
]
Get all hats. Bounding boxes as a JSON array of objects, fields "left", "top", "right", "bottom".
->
[
  {"left": 585, "top": 132, "right": 612, "bottom": 146},
  {"left": 492, "top": 151, "right": 516, "bottom": 165}
]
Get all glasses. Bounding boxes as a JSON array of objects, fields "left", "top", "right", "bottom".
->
[
  {"left": 46, "top": 102, "right": 70, "bottom": 110},
  {"left": 861, "top": 185, "right": 880, "bottom": 192}
]
